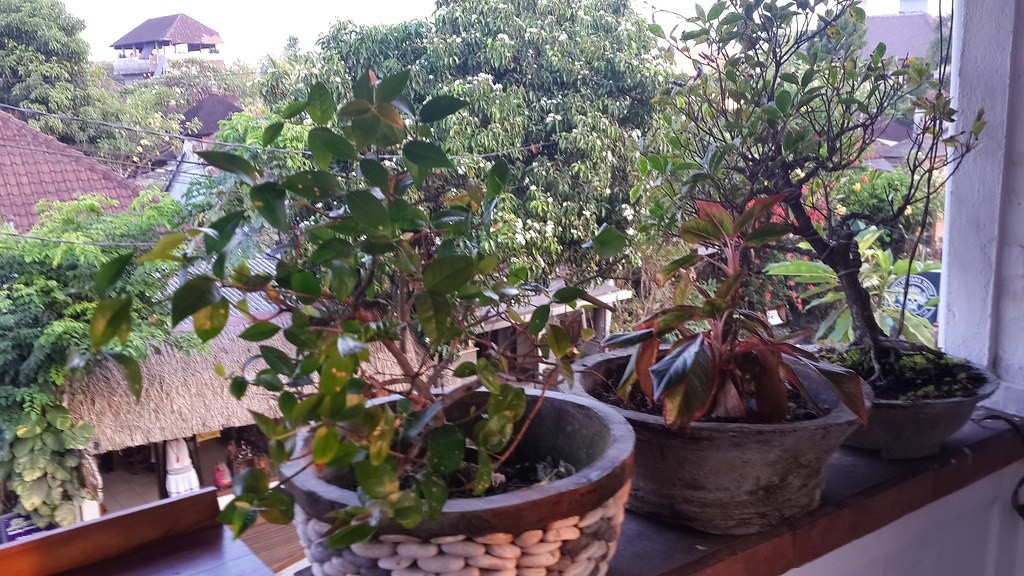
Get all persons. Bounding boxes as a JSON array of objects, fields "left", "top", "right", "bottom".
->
[
  {"left": 214, "top": 440, "right": 236, "bottom": 491},
  {"left": 81, "top": 453, "right": 106, "bottom": 514},
  {"left": 160, "top": 435, "right": 201, "bottom": 498},
  {"left": 254, "top": 452, "right": 273, "bottom": 477}
]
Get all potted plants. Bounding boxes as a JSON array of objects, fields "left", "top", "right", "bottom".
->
[
  {"left": 81, "top": 62, "right": 635, "bottom": 576},
  {"left": 631, "top": 0, "right": 1004, "bottom": 462},
  {"left": 562, "top": 172, "right": 877, "bottom": 544}
]
